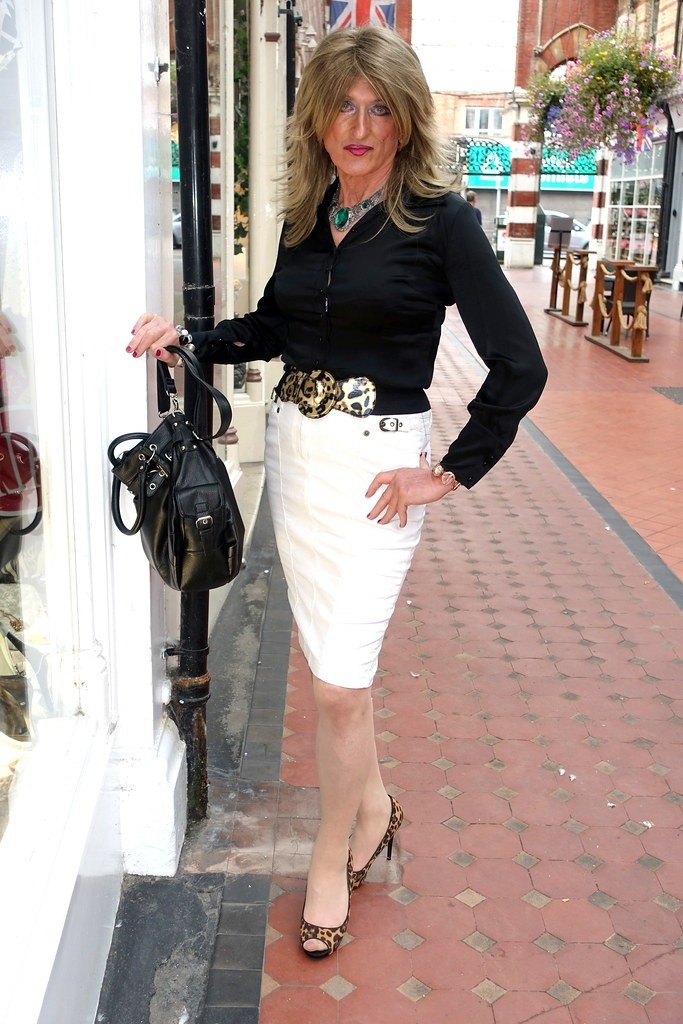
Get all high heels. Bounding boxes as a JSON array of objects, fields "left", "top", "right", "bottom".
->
[
  {"left": 349, "top": 793, "right": 403, "bottom": 890},
  {"left": 299, "top": 844, "right": 353, "bottom": 958}
]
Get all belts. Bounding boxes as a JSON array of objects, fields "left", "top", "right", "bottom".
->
[{"left": 273, "top": 367, "right": 431, "bottom": 419}]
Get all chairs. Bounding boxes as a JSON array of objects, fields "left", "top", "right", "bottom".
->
[{"left": 595, "top": 260, "right": 656, "bottom": 343}]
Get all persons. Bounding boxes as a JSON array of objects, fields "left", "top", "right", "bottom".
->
[
  {"left": 126, "top": 30, "right": 548, "bottom": 960},
  {"left": 0, "top": 312, "right": 16, "bottom": 359},
  {"left": 466, "top": 190, "right": 482, "bottom": 227}
]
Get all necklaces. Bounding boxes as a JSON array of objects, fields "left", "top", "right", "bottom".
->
[{"left": 327, "top": 181, "right": 388, "bottom": 232}]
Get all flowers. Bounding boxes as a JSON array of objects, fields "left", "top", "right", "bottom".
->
[{"left": 523, "top": 26, "right": 683, "bottom": 164}]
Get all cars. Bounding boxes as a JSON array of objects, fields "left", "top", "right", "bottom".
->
[{"left": 543, "top": 209, "right": 590, "bottom": 256}]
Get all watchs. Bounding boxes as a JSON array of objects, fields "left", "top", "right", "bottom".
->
[{"left": 432, "top": 463, "right": 461, "bottom": 491}]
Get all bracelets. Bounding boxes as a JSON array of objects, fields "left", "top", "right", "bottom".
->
[{"left": 169, "top": 324, "right": 195, "bottom": 368}]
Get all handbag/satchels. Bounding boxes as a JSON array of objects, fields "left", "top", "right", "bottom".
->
[{"left": 108, "top": 345, "right": 246, "bottom": 593}]
